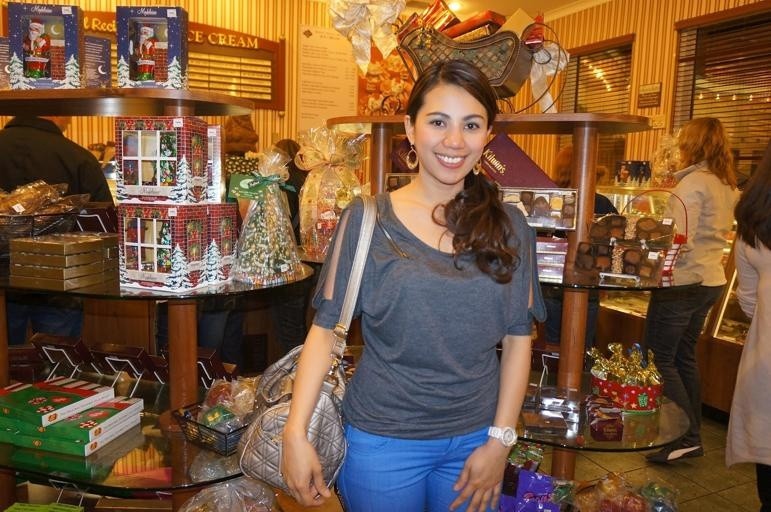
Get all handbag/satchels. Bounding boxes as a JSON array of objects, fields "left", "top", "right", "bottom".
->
[{"left": 239, "top": 340, "right": 348, "bottom": 501}]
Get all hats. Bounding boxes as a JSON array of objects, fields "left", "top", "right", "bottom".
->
[{"left": 275, "top": 139, "right": 300, "bottom": 159}]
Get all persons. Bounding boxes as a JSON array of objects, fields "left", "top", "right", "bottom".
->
[
  {"left": 546, "top": 165, "right": 620, "bottom": 372},
  {"left": 639, "top": 115, "right": 743, "bottom": 466},
  {"left": 196, "top": 114, "right": 311, "bottom": 378},
  {"left": 280, "top": 61, "right": 549, "bottom": 512},
  {"left": 725, "top": 142, "right": 771, "bottom": 512},
  {"left": 1, "top": 115, "right": 117, "bottom": 346}
]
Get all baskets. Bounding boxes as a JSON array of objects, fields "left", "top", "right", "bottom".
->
[
  {"left": 172, "top": 403, "right": 247, "bottom": 456},
  {"left": 0, "top": 212, "right": 81, "bottom": 256},
  {"left": 619, "top": 190, "right": 688, "bottom": 282}
]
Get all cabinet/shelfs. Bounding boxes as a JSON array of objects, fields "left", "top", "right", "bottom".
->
[
  {"left": 326, "top": 113, "right": 691, "bottom": 482},
  {"left": 0, "top": 87, "right": 314, "bottom": 512}
]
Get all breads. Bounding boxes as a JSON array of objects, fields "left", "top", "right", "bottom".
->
[{"left": 0, "top": 183, "right": 74, "bottom": 239}]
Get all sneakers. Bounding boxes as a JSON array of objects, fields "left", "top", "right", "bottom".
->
[{"left": 645, "top": 437, "right": 705, "bottom": 463}]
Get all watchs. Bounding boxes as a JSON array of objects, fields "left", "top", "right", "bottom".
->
[{"left": 488, "top": 424, "right": 519, "bottom": 448}]
